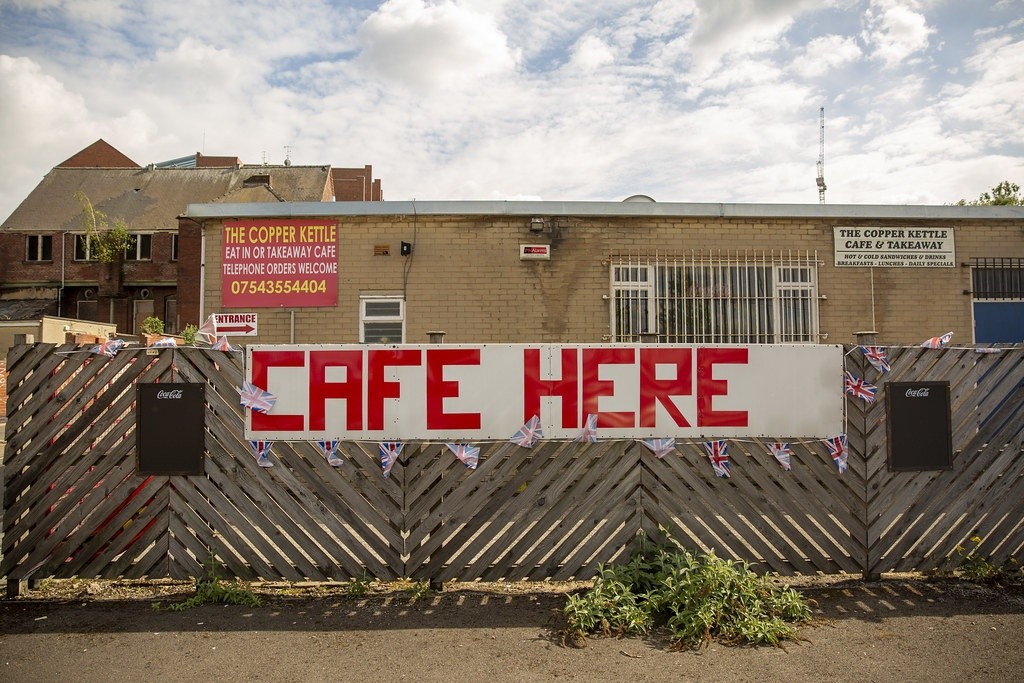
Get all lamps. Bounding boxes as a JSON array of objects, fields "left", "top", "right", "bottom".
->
[
  {"left": 399, "top": 241, "right": 411, "bottom": 258},
  {"left": 529, "top": 217, "right": 545, "bottom": 233}
]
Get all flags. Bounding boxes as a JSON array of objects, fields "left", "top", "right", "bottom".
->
[
  {"left": 91, "top": 316, "right": 952, "bottom": 478},
  {"left": 444, "top": 444, "right": 481, "bottom": 470}
]
